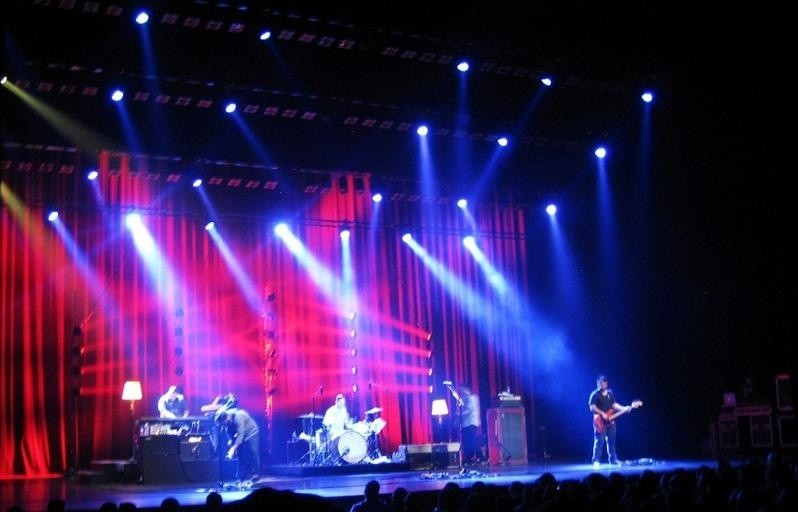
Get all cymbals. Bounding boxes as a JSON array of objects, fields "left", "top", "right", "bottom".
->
[
  {"left": 366, "top": 407, "right": 382, "bottom": 413},
  {"left": 299, "top": 415, "right": 324, "bottom": 419}
]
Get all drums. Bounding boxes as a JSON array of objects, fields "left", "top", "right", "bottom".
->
[{"left": 331, "top": 432, "right": 368, "bottom": 464}]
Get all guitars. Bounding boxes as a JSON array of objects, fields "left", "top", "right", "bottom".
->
[{"left": 594, "top": 400, "right": 643, "bottom": 433}]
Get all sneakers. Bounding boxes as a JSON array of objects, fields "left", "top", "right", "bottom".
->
[
  {"left": 609, "top": 459, "right": 622, "bottom": 465},
  {"left": 593, "top": 460, "right": 600, "bottom": 467}
]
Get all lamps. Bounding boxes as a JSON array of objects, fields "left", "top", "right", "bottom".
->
[
  {"left": 121, "top": 380, "right": 143, "bottom": 460},
  {"left": 431, "top": 399, "right": 449, "bottom": 444}
]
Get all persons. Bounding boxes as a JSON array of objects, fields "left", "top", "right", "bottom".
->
[
  {"left": 589, "top": 376, "right": 631, "bottom": 469},
  {"left": 158, "top": 385, "right": 191, "bottom": 419},
  {"left": 455, "top": 384, "right": 488, "bottom": 474},
  {"left": 216, "top": 407, "right": 261, "bottom": 485},
  {"left": 322, "top": 394, "right": 351, "bottom": 442},
  {"left": 48, "top": 450, "right": 797, "bottom": 511}
]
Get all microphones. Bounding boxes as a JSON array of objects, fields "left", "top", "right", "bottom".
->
[{"left": 442, "top": 381, "right": 452, "bottom": 386}]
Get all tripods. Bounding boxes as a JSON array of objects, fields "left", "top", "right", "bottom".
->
[{"left": 295, "top": 418, "right": 325, "bottom": 466}]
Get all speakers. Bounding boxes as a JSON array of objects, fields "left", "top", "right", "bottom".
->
[
  {"left": 716, "top": 373, "right": 797, "bottom": 452},
  {"left": 487, "top": 407, "right": 527, "bottom": 465},
  {"left": 139, "top": 431, "right": 242, "bottom": 486}
]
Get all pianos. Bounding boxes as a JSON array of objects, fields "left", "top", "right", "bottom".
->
[{"left": 133, "top": 419, "right": 216, "bottom": 434}]
